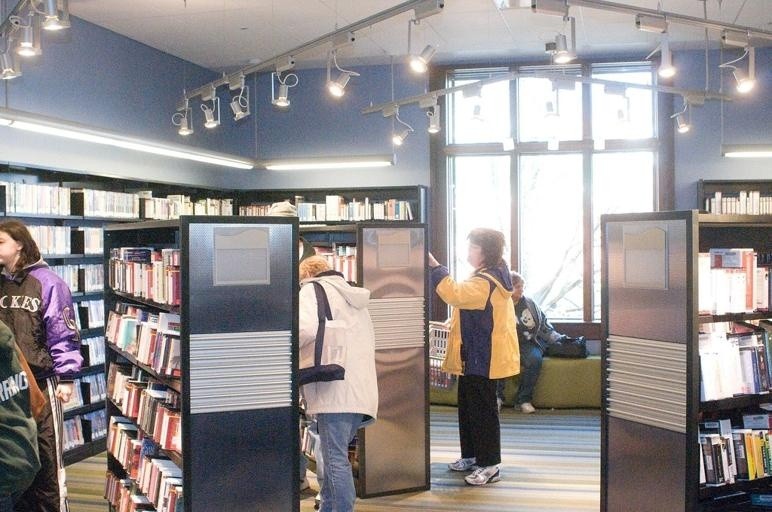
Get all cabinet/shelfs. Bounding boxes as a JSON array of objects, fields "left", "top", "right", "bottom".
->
[
  {"left": 601, "top": 209, "right": 771, "bottom": 511},
  {"left": 696, "top": 178, "right": 771, "bottom": 214},
  {"left": 299, "top": 224, "right": 430, "bottom": 499},
  {"left": 103, "top": 216, "right": 299, "bottom": 511},
  {"left": 237, "top": 185, "right": 429, "bottom": 224},
  {"left": 0, "top": 160, "right": 236, "bottom": 467}
]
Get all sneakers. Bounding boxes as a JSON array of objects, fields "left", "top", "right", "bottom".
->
[
  {"left": 515, "top": 403, "right": 536, "bottom": 414},
  {"left": 464, "top": 465, "right": 500, "bottom": 486},
  {"left": 448, "top": 457, "right": 479, "bottom": 471}
]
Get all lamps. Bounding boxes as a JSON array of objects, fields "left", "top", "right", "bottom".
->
[
  {"left": 1, "top": 106, "right": 13, "bottom": 126},
  {"left": 0, "top": 0, "right": 74, "bottom": 80},
  {"left": 720, "top": 144, "right": 772, "bottom": 160},
  {"left": 258, "top": 154, "right": 396, "bottom": 170},
  {"left": 7, "top": 109, "right": 258, "bottom": 169}
]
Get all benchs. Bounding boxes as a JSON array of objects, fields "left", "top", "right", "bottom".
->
[{"left": 429, "top": 354, "right": 602, "bottom": 408}]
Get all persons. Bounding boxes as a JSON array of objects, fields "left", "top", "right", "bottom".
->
[
  {"left": 268, "top": 200, "right": 317, "bottom": 264},
  {"left": 0, "top": 218, "right": 84, "bottom": 512},
  {"left": 294, "top": 255, "right": 379, "bottom": 512},
  {"left": 497, "top": 270, "right": 551, "bottom": 414},
  {"left": 0, "top": 321, "right": 58, "bottom": 511},
  {"left": 429, "top": 230, "right": 521, "bottom": 486}
]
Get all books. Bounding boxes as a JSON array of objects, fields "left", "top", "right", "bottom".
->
[
  {"left": 103, "top": 250, "right": 185, "bottom": 512},
  {"left": 239, "top": 194, "right": 416, "bottom": 223},
  {"left": 25, "top": 222, "right": 111, "bottom": 447},
  {"left": 313, "top": 244, "right": 358, "bottom": 284},
  {"left": 2, "top": 179, "right": 234, "bottom": 219},
  {"left": 299, "top": 408, "right": 359, "bottom": 480},
  {"left": 697, "top": 185, "right": 771, "bottom": 511}
]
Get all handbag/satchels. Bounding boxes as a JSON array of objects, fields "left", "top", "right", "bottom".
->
[{"left": 547, "top": 335, "right": 586, "bottom": 358}]
